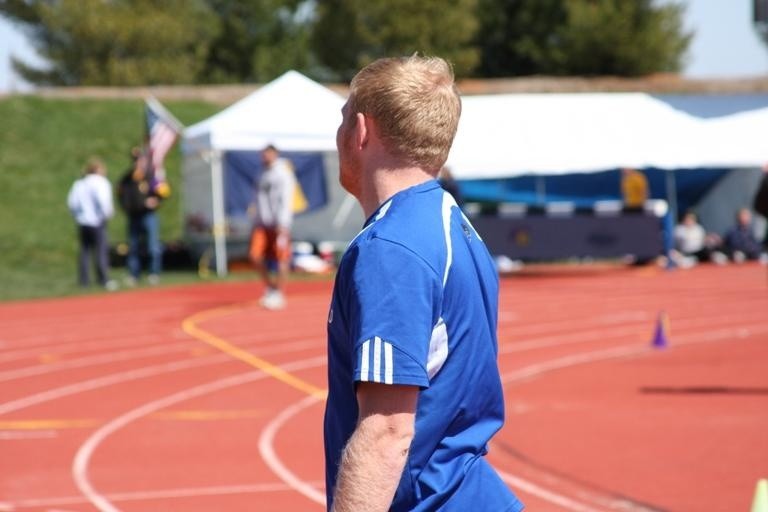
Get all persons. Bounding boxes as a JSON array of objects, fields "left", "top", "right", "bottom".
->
[
  {"left": 754, "top": 159, "right": 768, "bottom": 218},
  {"left": 116, "top": 144, "right": 162, "bottom": 287},
  {"left": 653, "top": 210, "right": 709, "bottom": 269},
  {"left": 620, "top": 166, "right": 647, "bottom": 215},
  {"left": 325, "top": 46, "right": 523, "bottom": 512},
  {"left": 66, "top": 153, "right": 114, "bottom": 289},
  {"left": 710, "top": 208, "right": 764, "bottom": 265},
  {"left": 247, "top": 146, "right": 296, "bottom": 312}
]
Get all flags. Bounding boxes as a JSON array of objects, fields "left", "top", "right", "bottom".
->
[{"left": 141, "top": 101, "right": 179, "bottom": 182}]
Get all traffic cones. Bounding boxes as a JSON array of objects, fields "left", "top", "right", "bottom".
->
[{"left": 652, "top": 309, "right": 672, "bottom": 347}]
